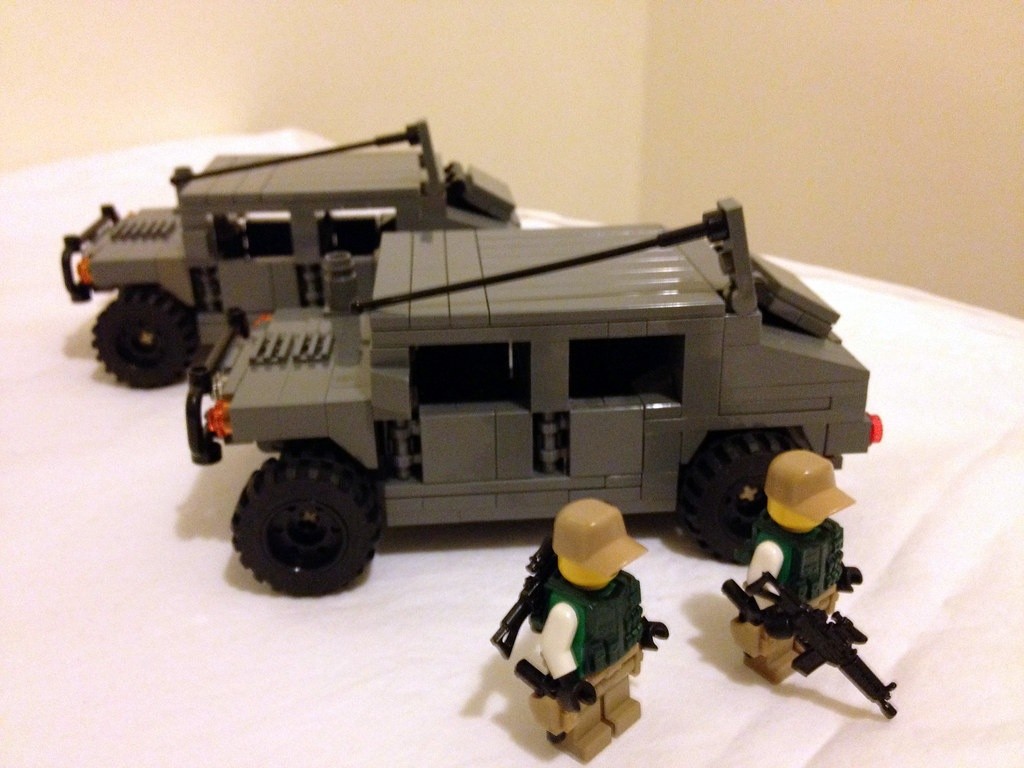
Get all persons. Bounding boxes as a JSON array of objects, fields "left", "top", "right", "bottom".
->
[
  {"left": 722, "top": 448, "right": 898, "bottom": 720},
  {"left": 491, "top": 499, "right": 670, "bottom": 764}
]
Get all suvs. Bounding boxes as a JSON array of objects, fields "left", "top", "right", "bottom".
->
[
  {"left": 60, "top": 118, "right": 520, "bottom": 393},
  {"left": 185, "top": 193, "right": 881, "bottom": 600}
]
